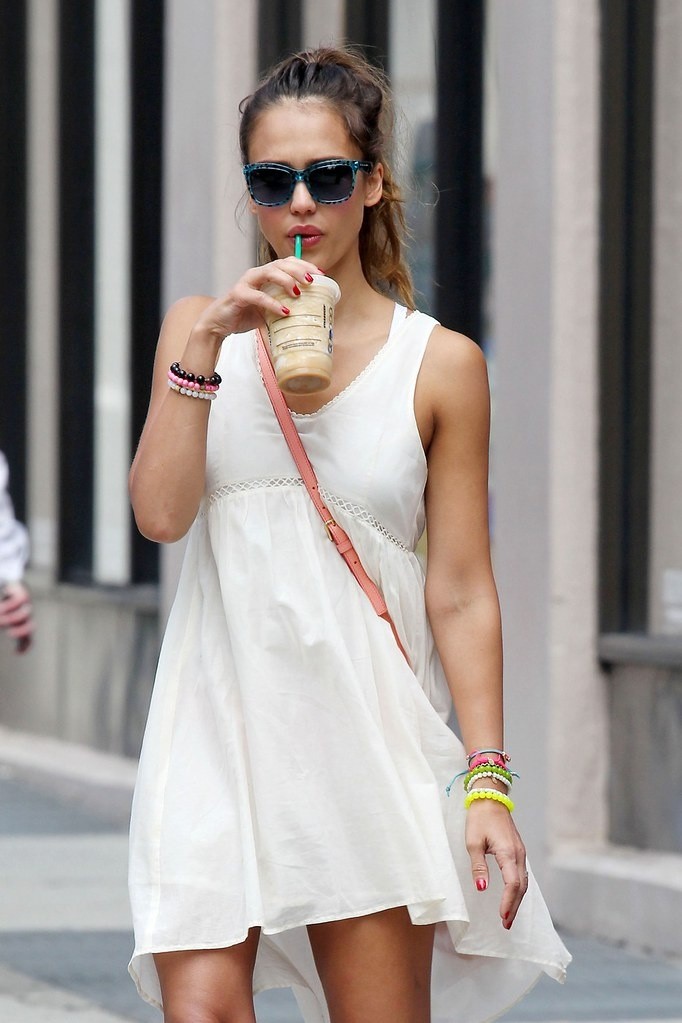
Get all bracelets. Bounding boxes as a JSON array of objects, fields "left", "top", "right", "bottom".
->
[
  {"left": 167, "top": 361, "right": 222, "bottom": 400},
  {"left": 446, "top": 749, "right": 521, "bottom": 813}
]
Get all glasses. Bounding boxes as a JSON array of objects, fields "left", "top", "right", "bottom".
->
[{"left": 243, "top": 158, "right": 373, "bottom": 208}]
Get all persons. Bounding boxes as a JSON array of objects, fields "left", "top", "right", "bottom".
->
[
  {"left": 124, "top": 42, "right": 576, "bottom": 1023},
  {"left": 0, "top": 448, "right": 40, "bottom": 658}
]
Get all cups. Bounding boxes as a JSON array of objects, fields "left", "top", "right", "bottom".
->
[{"left": 259, "top": 273, "right": 342, "bottom": 396}]
